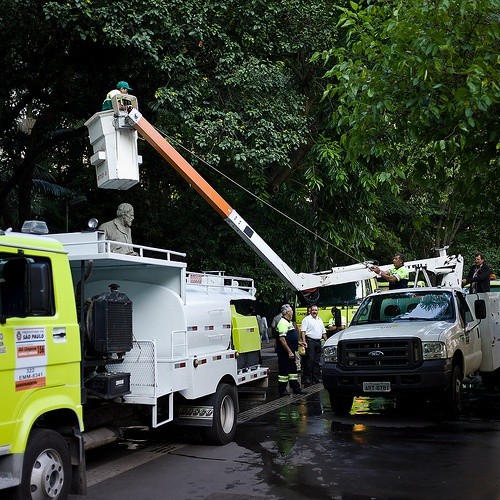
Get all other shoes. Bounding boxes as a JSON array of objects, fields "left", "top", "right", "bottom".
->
[
  {"left": 279, "top": 389, "right": 289, "bottom": 395},
  {"left": 294, "top": 389, "right": 308, "bottom": 394}
]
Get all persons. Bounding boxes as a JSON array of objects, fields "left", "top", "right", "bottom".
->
[
  {"left": 98, "top": 202, "right": 139, "bottom": 256},
  {"left": 259, "top": 313, "right": 270, "bottom": 344},
  {"left": 103, "top": 80, "right": 133, "bottom": 112},
  {"left": 368, "top": 254, "right": 409, "bottom": 290},
  {"left": 328, "top": 306, "right": 346, "bottom": 336},
  {"left": 461, "top": 253, "right": 490, "bottom": 294},
  {"left": 301, "top": 305, "right": 328, "bottom": 386},
  {"left": 274, "top": 307, "right": 309, "bottom": 395}
]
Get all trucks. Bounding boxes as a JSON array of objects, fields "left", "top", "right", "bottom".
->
[
  {"left": 0, "top": 218, "right": 269, "bottom": 500},
  {"left": 295, "top": 278, "right": 426, "bottom": 365}
]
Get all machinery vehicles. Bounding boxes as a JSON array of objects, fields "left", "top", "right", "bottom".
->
[{"left": 84, "top": 94, "right": 500, "bottom": 418}]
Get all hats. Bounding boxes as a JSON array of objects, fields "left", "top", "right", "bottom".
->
[{"left": 117, "top": 81, "right": 133, "bottom": 91}]
